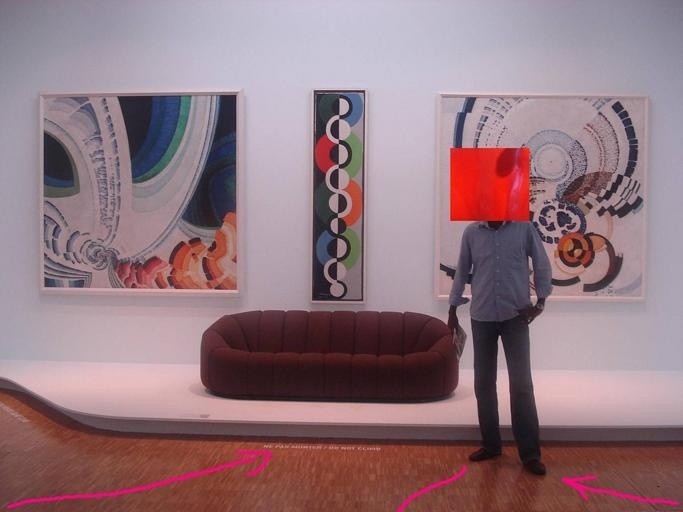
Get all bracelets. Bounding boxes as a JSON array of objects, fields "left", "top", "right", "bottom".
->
[{"left": 535, "top": 303, "right": 545, "bottom": 312}]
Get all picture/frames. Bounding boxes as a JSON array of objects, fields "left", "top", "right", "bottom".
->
[
  {"left": 308, "top": 88, "right": 367, "bottom": 306},
  {"left": 33, "top": 89, "right": 247, "bottom": 302},
  {"left": 434, "top": 92, "right": 649, "bottom": 304}
]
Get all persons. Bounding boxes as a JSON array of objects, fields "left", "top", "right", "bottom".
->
[{"left": 447, "top": 222, "right": 553, "bottom": 476}]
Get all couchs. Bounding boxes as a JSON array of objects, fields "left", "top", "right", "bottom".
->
[{"left": 199, "top": 310, "right": 460, "bottom": 401}]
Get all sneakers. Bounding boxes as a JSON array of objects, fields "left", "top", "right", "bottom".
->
[
  {"left": 470, "top": 448, "right": 495, "bottom": 461},
  {"left": 528, "top": 460, "right": 545, "bottom": 475}
]
